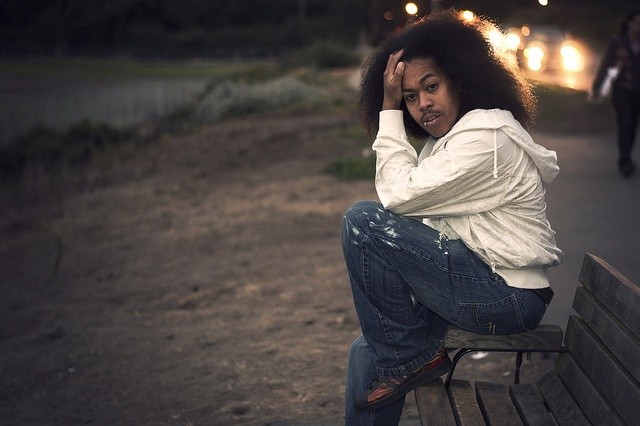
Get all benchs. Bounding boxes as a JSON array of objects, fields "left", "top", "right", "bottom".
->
[{"left": 414, "top": 253, "right": 638, "bottom": 424}]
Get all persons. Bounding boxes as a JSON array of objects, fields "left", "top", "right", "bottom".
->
[
  {"left": 590, "top": 11, "right": 640, "bottom": 177},
  {"left": 341, "top": 8, "right": 564, "bottom": 426}
]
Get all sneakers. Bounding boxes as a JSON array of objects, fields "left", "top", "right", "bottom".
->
[{"left": 353, "top": 347, "right": 453, "bottom": 413}]
